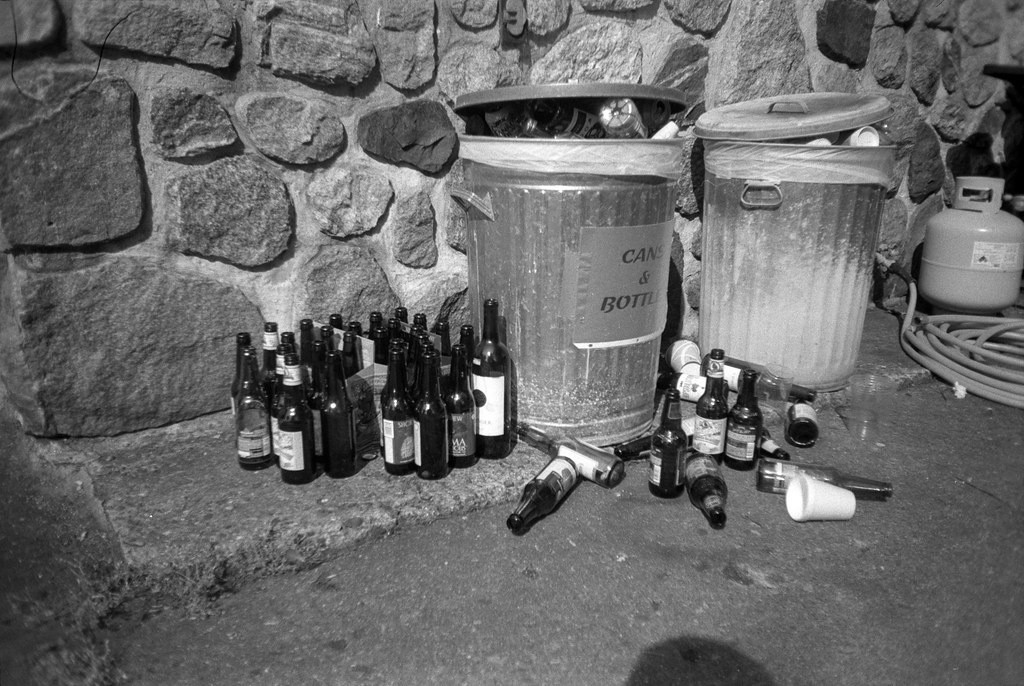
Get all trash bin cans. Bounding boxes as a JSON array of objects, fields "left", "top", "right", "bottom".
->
[
  {"left": 451, "top": 85, "right": 687, "bottom": 448},
  {"left": 693, "top": 92, "right": 898, "bottom": 392}
]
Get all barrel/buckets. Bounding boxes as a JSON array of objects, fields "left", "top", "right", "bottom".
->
[
  {"left": 440, "top": 79, "right": 688, "bottom": 447},
  {"left": 688, "top": 90, "right": 896, "bottom": 395}
]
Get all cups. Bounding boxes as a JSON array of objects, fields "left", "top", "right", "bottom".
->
[
  {"left": 784, "top": 472, "right": 856, "bottom": 523},
  {"left": 846, "top": 372, "right": 895, "bottom": 439},
  {"left": 752, "top": 364, "right": 793, "bottom": 433}
]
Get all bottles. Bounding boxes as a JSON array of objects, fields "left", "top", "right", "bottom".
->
[
  {"left": 229, "top": 297, "right": 513, "bottom": 487},
  {"left": 614, "top": 344, "right": 894, "bottom": 527},
  {"left": 515, "top": 421, "right": 624, "bottom": 489},
  {"left": 460, "top": 95, "right": 672, "bottom": 139},
  {"left": 506, "top": 455, "right": 580, "bottom": 533}
]
